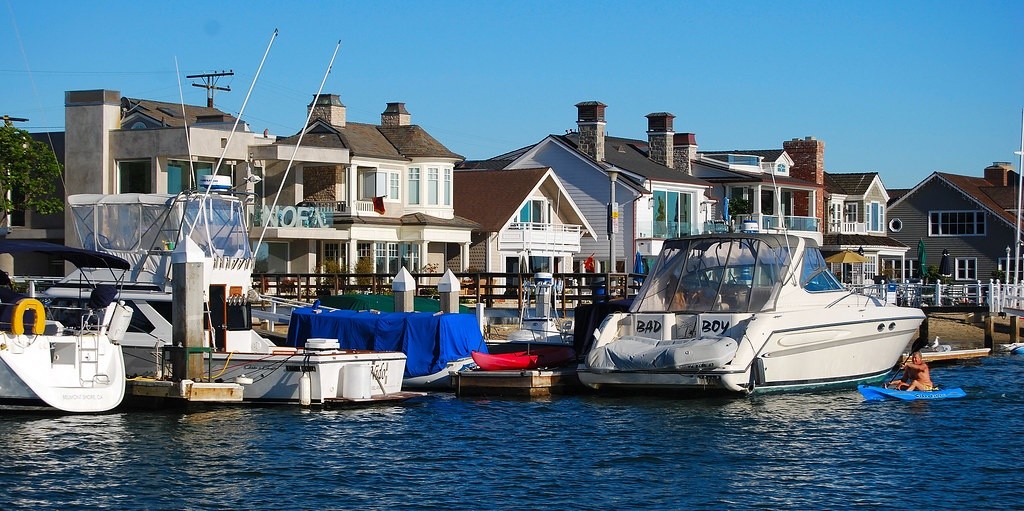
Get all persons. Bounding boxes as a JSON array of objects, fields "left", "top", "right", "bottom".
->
[
  {"left": 888, "top": 351, "right": 933, "bottom": 391},
  {"left": 633, "top": 252, "right": 644, "bottom": 291},
  {"left": 665, "top": 275, "right": 686, "bottom": 311}
]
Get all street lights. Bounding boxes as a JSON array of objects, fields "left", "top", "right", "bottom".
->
[
  {"left": 1005, "top": 246, "right": 1011, "bottom": 284},
  {"left": 602, "top": 165, "right": 622, "bottom": 295}
]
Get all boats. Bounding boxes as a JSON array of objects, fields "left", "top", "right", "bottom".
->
[
  {"left": 483, "top": 272, "right": 575, "bottom": 362},
  {"left": 0, "top": 237, "right": 134, "bottom": 412},
  {"left": 857, "top": 385, "right": 967, "bottom": 401},
  {"left": 471, "top": 347, "right": 576, "bottom": 371},
  {"left": 576, "top": 220, "right": 927, "bottom": 395},
  {"left": 46, "top": 189, "right": 427, "bottom": 409},
  {"left": 285, "top": 300, "right": 489, "bottom": 388}
]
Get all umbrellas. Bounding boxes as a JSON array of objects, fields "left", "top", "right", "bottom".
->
[
  {"left": 917, "top": 238, "right": 927, "bottom": 278},
  {"left": 723, "top": 197, "right": 728, "bottom": 225},
  {"left": 939, "top": 248, "right": 951, "bottom": 284},
  {"left": 824, "top": 248, "right": 873, "bottom": 279}
]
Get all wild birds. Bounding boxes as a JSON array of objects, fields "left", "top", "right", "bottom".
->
[{"left": 924, "top": 335, "right": 941, "bottom": 352}]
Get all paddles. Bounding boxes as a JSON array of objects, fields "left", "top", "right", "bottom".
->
[{"left": 882, "top": 335, "right": 929, "bottom": 389}]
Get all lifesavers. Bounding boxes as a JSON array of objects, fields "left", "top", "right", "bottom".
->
[{"left": 9, "top": 298, "right": 46, "bottom": 336}]
